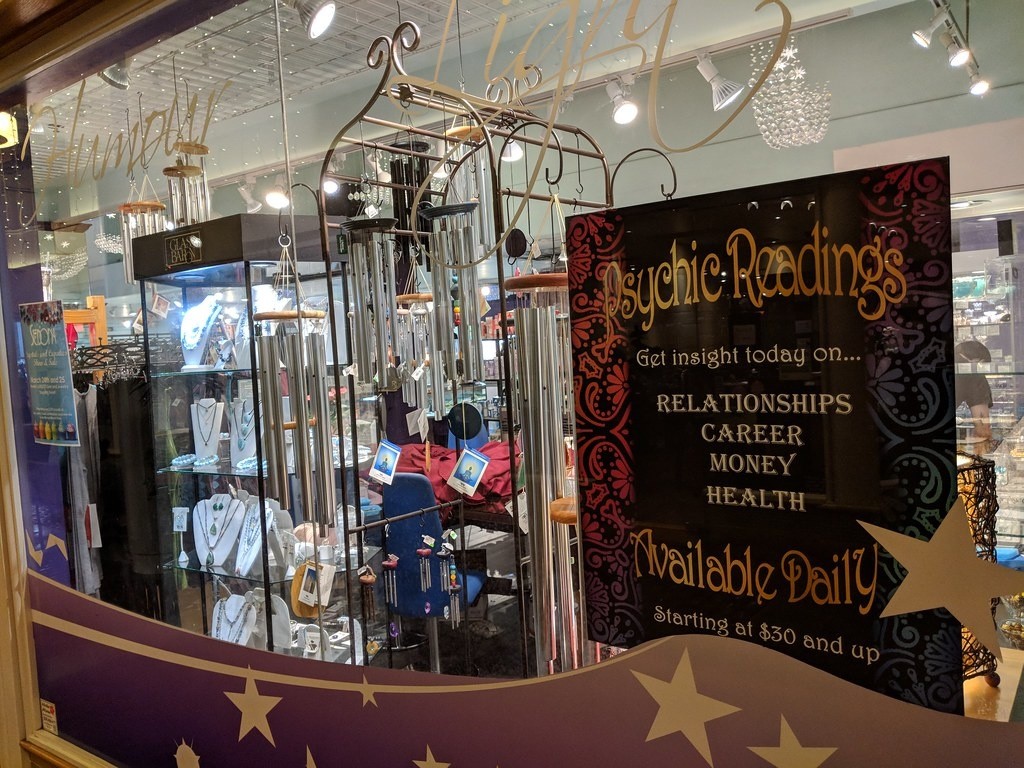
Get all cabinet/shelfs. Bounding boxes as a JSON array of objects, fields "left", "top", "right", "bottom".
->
[
  {"left": 948, "top": 270, "right": 1024, "bottom": 553},
  {"left": 132, "top": 211, "right": 369, "bottom": 671}
]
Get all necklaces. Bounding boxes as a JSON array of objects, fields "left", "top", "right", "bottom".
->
[
  {"left": 196, "top": 493, "right": 245, "bottom": 567},
  {"left": 182, "top": 303, "right": 221, "bottom": 351},
  {"left": 239, "top": 295, "right": 282, "bottom": 345},
  {"left": 233, "top": 398, "right": 269, "bottom": 452},
  {"left": 249, "top": 594, "right": 275, "bottom": 614},
  {"left": 237, "top": 501, "right": 273, "bottom": 573},
  {"left": 215, "top": 597, "right": 254, "bottom": 644},
  {"left": 197, "top": 402, "right": 217, "bottom": 446}
]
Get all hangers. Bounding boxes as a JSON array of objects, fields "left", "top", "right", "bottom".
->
[{"left": 74, "top": 355, "right": 219, "bottom": 393}]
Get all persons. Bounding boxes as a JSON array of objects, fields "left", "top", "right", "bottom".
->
[{"left": 954, "top": 340, "right": 994, "bottom": 454}]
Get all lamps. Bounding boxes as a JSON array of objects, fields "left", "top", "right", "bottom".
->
[
  {"left": 237, "top": 184, "right": 263, "bottom": 213},
  {"left": 281, "top": 0, "right": 337, "bottom": 40},
  {"left": 266, "top": 174, "right": 289, "bottom": 209},
  {"left": 366, "top": 154, "right": 392, "bottom": 183},
  {"left": 966, "top": 63, "right": 989, "bottom": 95},
  {"left": 540, "top": 105, "right": 563, "bottom": 143},
  {"left": 697, "top": 54, "right": 745, "bottom": 111},
  {"left": 431, "top": 138, "right": 452, "bottom": 178},
  {"left": 938, "top": 32, "right": 970, "bottom": 68},
  {"left": 911, "top": 11, "right": 949, "bottom": 48},
  {"left": 325, "top": 161, "right": 339, "bottom": 193},
  {"left": 502, "top": 128, "right": 523, "bottom": 163},
  {"left": 101, "top": 56, "right": 135, "bottom": 90},
  {"left": 605, "top": 82, "right": 638, "bottom": 124}
]
